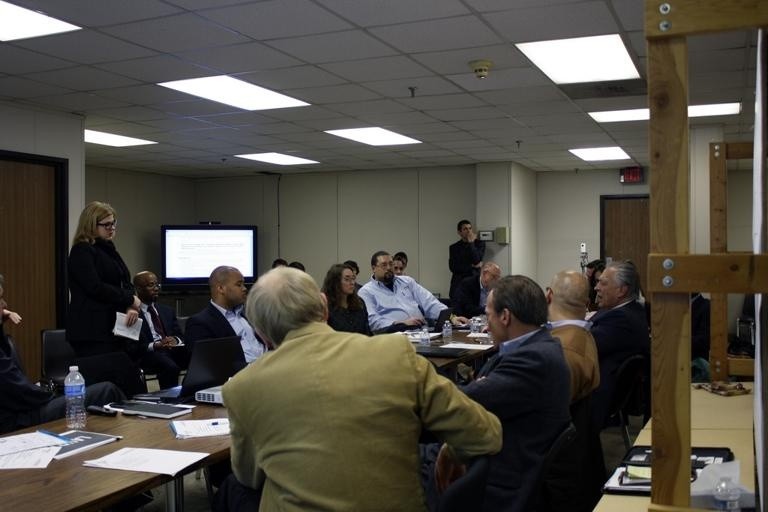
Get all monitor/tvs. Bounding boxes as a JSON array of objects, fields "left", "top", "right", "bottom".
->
[{"left": 161, "top": 224, "right": 258, "bottom": 284}]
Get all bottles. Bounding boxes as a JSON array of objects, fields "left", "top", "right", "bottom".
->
[
  {"left": 469, "top": 316, "right": 480, "bottom": 338},
  {"left": 418, "top": 328, "right": 432, "bottom": 346},
  {"left": 711, "top": 475, "right": 741, "bottom": 512},
  {"left": 64, "top": 365, "right": 87, "bottom": 430}
]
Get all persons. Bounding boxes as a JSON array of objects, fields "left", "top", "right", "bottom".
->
[
  {"left": 271, "top": 250, "right": 450, "bottom": 336},
  {"left": 449, "top": 219, "right": 486, "bottom": 321},
  {"left": 222, "top": 266, "right": 502, "bottom": 512},
  {"left": 480, "top": 262, "right": 501, "bottom": 311},
  {"left": 421, "top": 275, "right": 572, "bottom": 512},
  {"left": 132, "top": 270, "right": 191, "bottom": 391},
  {"left": 2, "top": 300, "right": 128, "bottom": 435},
  {"left": 61, "top": 199, "right": 143, "bottom": 402},
  {"left": 546, "top": 257, "right": 711, "bottom": 427},
  {"left": 185, "top": 265, "right": 273, "bottom": 377}
]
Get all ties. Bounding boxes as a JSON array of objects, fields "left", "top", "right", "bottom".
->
[{"left": 147, "top": 306, "right": 165, "bottom": 338}]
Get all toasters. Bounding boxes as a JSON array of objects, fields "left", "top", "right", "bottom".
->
[{"left": 443, "top": 320, "right": 453, "bottom": 342}]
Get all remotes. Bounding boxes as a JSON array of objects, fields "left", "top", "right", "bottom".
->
[{"left": 88, "top": 406, "right": 118, "bottom": 417}]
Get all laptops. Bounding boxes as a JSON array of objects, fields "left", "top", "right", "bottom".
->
[
  {"left": 413, "top": 308, "right": 453, "bottom": 333},
  {"left": 133, "top": 335, "right": 241, "bottom": 404}
]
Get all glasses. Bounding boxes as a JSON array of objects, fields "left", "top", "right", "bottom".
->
[
  {"left": 98, "top": 220, "right": 116, "bottom": 229},
  {"left": 139, "top": 283, "right": 160, "bottom": 288}
]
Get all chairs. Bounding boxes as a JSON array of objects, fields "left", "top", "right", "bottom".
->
[
  {"left": 427, "top": 351, "right": 650, "bottom": 512},
  {"left": 41, "top": 328, "right": 73, "bottom": 386}
]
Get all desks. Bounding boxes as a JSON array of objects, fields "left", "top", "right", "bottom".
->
[
  {"left": 591, "top": 377, "right": 758, "bottom": 511},
  {"left": 1, "top": 324, "right": 497, "bottom": 511}
]
